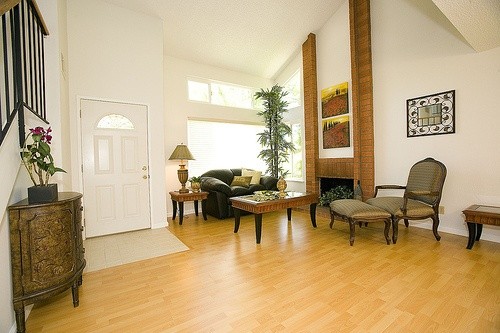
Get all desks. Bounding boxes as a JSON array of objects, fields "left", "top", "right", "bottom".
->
[
  {"left": 169, "top": 191, "right": 209, "bottom": 225},
  {"left": 229, "top": 191, "right": 319, "bottom": 244}
]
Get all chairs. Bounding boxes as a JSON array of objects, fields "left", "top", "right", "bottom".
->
[{"left": 365, "top": 158, "right": 447, "bottom": 244}]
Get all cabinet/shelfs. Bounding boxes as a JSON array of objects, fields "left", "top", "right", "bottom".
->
[{"left": 7, "top": 192, "right": 87, "bottom": 333}]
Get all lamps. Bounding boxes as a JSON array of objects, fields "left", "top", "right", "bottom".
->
[{"left": 168, "top": 142, "right": 195, "bottom": 193}]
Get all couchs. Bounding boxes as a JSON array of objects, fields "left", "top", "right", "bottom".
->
[{"left": 199, "top": 168, "right": 279, "bottom": 220}]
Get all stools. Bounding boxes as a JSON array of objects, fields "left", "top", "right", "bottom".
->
[
  {"left": 461, "top": 205, "right": 500, "bottom": 250},
  {"left": 329, "top": 199, "right": 391, "bottom": 246}
]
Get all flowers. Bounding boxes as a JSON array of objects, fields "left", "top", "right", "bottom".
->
[{"left": 22, "top": 126, "right": 67, "bottom": 186}]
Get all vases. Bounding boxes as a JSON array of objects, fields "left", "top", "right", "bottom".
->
[
  {"left": 277, "top": 176, "right": 288, "bottom": 197},
  {"left": 28, "top": 183, "right": 58, "bottom": 204}
]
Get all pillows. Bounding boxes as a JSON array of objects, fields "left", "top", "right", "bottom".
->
[
  {"left": 230, "top": 176, "right": 253, "bottom": 188},
  {"left": 242, "top": 169, "right": 262, "bottom": 185}
]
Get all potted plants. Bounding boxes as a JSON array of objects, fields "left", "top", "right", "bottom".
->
[{"left": 188, "top": 176, "right": 205, "bottom": 193}]
